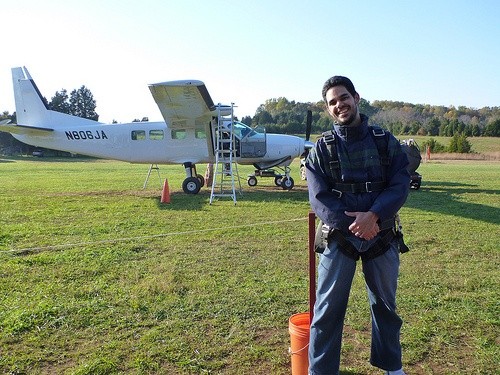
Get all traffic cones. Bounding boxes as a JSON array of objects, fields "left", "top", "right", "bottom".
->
[{"left": 160, "top": 178, "right": 172, "bottom": 204}]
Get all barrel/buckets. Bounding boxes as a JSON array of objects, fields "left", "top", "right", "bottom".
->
[{"left": 288, "top": 311, "right": 316, "bottom": 375}]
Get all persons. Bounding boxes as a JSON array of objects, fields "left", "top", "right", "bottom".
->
[{"left": 303, "top": 75, "right": 411, "bottom": 375}]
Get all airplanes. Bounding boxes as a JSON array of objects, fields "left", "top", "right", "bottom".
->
[{"left": 0, "top": 64, "right": 318, "bottom": 195}]
